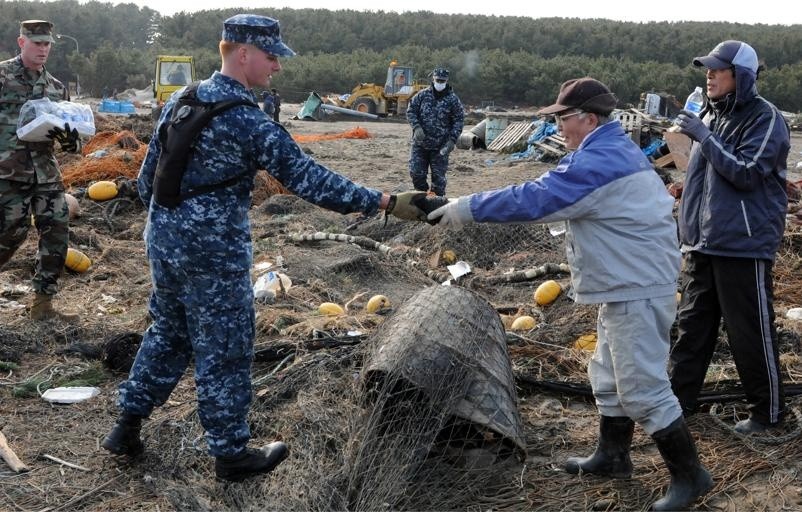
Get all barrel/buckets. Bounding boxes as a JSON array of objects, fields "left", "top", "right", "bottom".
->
[
  {"left": 471, "top": 119, "right": 486, "bottom": 138},
  {"left": 98, "top": 97, "right": 135, "bottom": 113},
  {"left": 362, "top": 284, "right": 530, "bottom": 469},
  {"left": 484, "top": 116, "right": 509, "bottom": 148},
  {"left": 455, "top": 130, "right": 478, "bottom": 148}
]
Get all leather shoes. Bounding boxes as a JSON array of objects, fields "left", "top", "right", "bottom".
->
[{"left": 735, "top": 418, "right": 783, "bottom": 434}]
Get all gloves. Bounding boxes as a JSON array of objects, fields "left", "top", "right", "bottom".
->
[
  {"left": 676, "top": 110, "right": 712, "bottom": 143},
  {"left": 427, "top": 194, "right": 473, "bottom": 233},
  {"left": 445, "top": 140, "right": 454, "bottom": 152},
  {"left": 385, "top": 191, "right": 426, "bottom": 221},
  {"left": 414, "top": 127, "right": 425, "bottom": 141},
  {"left": 46, "top": 123, "right": 79, "bottom": 151}
]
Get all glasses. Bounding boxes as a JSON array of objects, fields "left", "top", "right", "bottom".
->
[{"left": 555, "top": 110, "right": 585, "bottom": 123}]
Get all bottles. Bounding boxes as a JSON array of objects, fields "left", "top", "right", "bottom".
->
[
  {"left": 679, "top": 87, "right": 703, "bottom": 130},
  {"left": 58, "top": 102, "right": 95, "bottom": 123},
  {"left": 440, "top": 147, "right": 448, "bottom": 156}
]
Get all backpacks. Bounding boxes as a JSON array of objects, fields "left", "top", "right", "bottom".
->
[{"left": 151, "top": 79, "right": 259, "bottom": 206}]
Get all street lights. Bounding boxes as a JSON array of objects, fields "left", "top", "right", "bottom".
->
[{"left": 56, "top": 33, "right": 80, "bottom": 98}]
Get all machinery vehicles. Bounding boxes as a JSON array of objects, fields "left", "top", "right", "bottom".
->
[
  {"left": 321, "top": 60, "right": 433, "bottom": 122},
  {"left": 146, "top": 55, "right": 198, "bottom": 124}
]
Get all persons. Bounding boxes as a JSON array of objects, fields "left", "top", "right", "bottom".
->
[
  {"left": 260, "top": 88, "right": 280, "bottom": 122},
  {"left": 0, "top": 20, "right": 81, "bottom": 325},
  {"left": 408, "top": 69, "right": 463, "bottom": 197},
  {"left": 427, "top": 78, "right": 716, "bottom": 511},
  {"left": 667, "top": 39, "right": 791, "bottom": 435},
  {"left": 101, "top": 85, "right": 118, "bottom": 100},
  {"left": 167, "top": 64, "right": 186, "bottom": 86},
  {"left": 100, "top": 13, "right": 436, "bottom": 481}
]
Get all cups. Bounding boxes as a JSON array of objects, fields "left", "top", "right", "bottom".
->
[{"left": 547, "top": 220, "right": 566, "bottom": 238}]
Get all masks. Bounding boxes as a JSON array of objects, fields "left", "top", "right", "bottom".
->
[{"left": 433, "top": 79, "right": 447, "bottom": 92}]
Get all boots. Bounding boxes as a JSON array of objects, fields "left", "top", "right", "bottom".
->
[
  {"left": 567, "top": 415, "right": 635, "bottom": 478},
  {"left": 101, "top": 412, "right": 145, "bottom": 457},
  {"left": 215, "top": 441, "right": 289, "bottom": 483},
  {"left": 30, "top": 294, "right": 80, "bottom": 322},
  {"left": 650, "top": 414, "right": 712, "bottom": 512}
]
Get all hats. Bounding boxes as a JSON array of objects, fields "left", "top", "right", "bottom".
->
[
  {"left": 223, "top": 14, "right": 296, "bottom": 57},
  {"left": 539, "top": 78, "right": 616, "bottom": 114},
  {"left": 20, "top": 20, "right": 56, "bottom": 44},
  {"left": 433, "top": 68, "right": 449, "bottom": 80}
]
